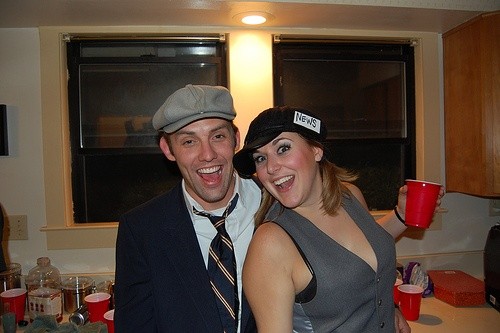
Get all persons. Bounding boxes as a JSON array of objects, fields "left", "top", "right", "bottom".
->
[
  {"left": 114, "top": 84, "right": 266, "bottom": 333},
  {"left": 233, "top": 107, "right": 449, "bottom": 333}
]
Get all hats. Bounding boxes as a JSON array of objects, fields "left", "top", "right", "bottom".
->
[
  {"left": 152, "top": 84, "right": 237, "bottom": 134},
  {"left": 233, "top": 106, "right": 329, "bottom": 175}
]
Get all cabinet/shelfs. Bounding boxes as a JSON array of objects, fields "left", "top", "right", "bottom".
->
[{"left": 442, "top": 12, "right": 500, "bottom": 198}]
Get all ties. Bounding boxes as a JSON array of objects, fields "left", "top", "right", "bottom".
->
[{"left": 193, "top": 193, "right": 240, "bottom": 333}]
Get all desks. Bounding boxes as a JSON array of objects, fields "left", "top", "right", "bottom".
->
[{"left": 395, "top": 284, "right": 500, "bottom": 333}]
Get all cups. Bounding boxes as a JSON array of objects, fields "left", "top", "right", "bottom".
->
[
  {"left": 397, "top": 284, "right": 424, "bottom": 321},
  {"left": 0, "top": 263, "right": 21, "bottom": 291},
  {"left": 393, "top": 279, "right": 403, "bottom": 304},
  {"left": 84, "top": 293, "right": 110, "bottom": 324},
  {"left": 104, "top": 309, "right": 114, "bottom": 333},
  {"left": 405, "top": 179, "right": 443, "bottom": 229},
  {"left": 0, "top": 288, "right": 27, "bottom": 325}
]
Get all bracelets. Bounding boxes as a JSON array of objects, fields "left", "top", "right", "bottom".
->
[{"left": 395, "top": 206, "right": 409, "bottom": 227}]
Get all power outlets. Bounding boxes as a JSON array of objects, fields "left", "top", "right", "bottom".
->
[{"left": 7, "top": 215, "right": 28, "bottom": 238}]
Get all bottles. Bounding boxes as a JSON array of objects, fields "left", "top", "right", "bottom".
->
[{"left": 28, "top": 257, "right": 63, "bottom": 323}]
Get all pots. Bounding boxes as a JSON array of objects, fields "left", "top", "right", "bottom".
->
[{"left": 63, "top": 277, "right": 95, "bottom": 314}]
